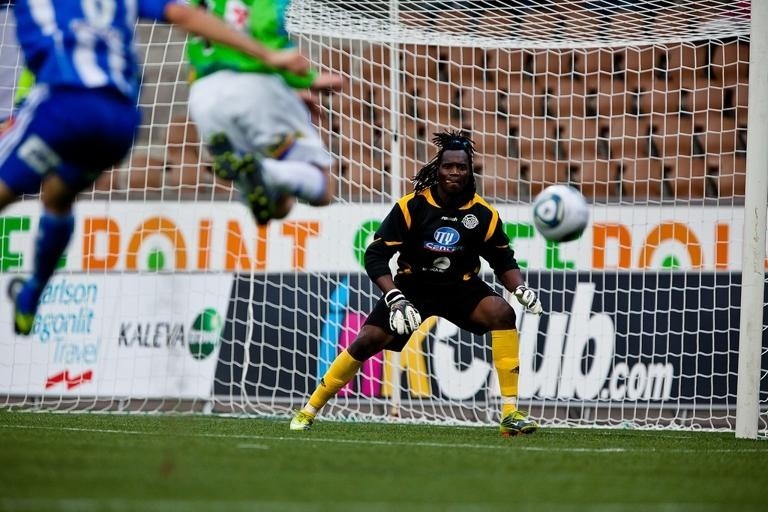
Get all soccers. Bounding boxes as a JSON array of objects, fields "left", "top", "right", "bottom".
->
[{"left": 532, "top": 183, "right": 588, "bottom": 244}]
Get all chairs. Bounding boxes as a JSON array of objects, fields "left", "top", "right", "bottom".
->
[{"left": 85, "top": 1, "right": 753, "bottom": 202}]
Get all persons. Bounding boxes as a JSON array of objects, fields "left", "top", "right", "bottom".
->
[
  {"left": 0, "top": 58, "right": 40, "bottom": 139},
  {"left": 179, "top": 0, "right": 338, "bottom": 227},
  {"left": 288, "top": 130, "right": 544, "bottom": 435},
  {"left": 0, "top": 0, "right": 313, "bottom": 337}
]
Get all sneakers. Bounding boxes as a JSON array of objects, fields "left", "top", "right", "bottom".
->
[
  {"left": 289, "top": 408, "right": 316, "bottom": 430},
  {"left": 500, "top": 410, "right": 538, "bottom": 436},
  {"left": 206, "top": 130, "right": 243, "bottom": 180},
  {"left": 8, "top": 277, "right": 34, "bottom": 332},
  {"left": 235, "top": 152, "right": 276, "bottom": 225}
]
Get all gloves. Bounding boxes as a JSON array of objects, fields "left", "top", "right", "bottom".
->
[
  {"left": 384, "top": 288, "right": 421, "bottom": 335},
  {"left": 511, "top": 284, "right": 543, "bottom": 316}
]
